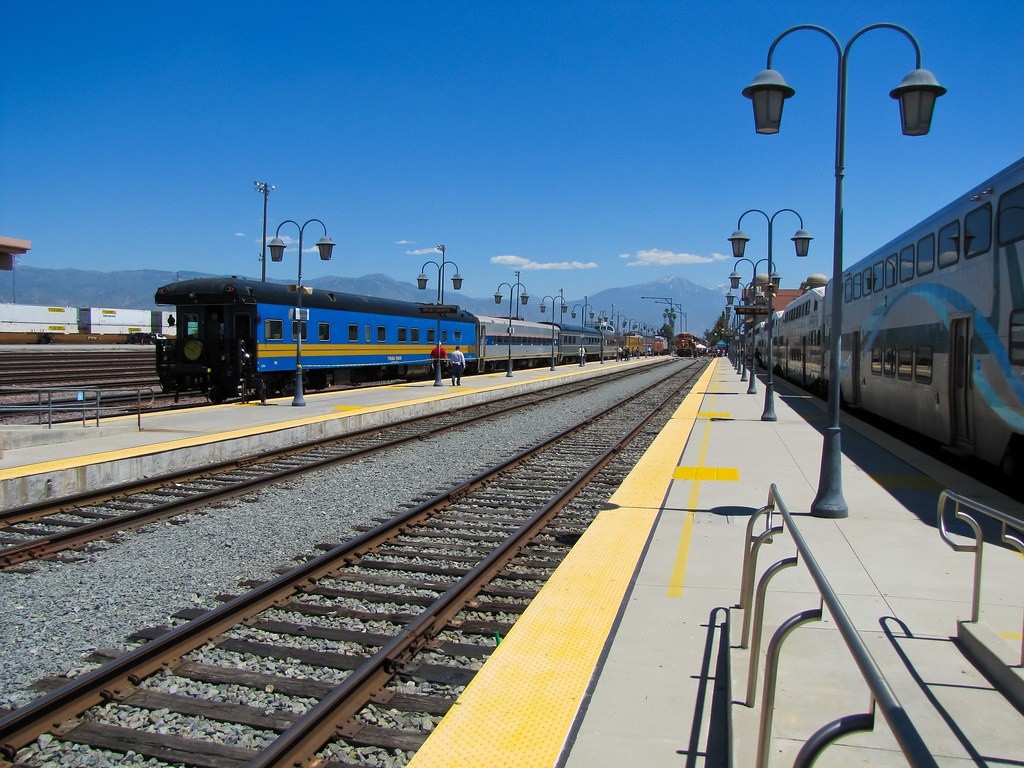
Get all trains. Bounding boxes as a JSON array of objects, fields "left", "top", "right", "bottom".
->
[
  {"left": 676, "top": 332, "right": 710, "bottom": 356},
  {"left": 151, "top": 274, "right": 671, "bottom": 404},
  {"left": 754, "top": 157, "right": 1024, "bottom": 491}
]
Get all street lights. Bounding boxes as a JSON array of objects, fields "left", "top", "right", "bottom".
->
[
  {"left": 538, "top": 295, "right": 571, "bottom": 373},
  {"left": 253, "top": 180, "right": 276, "bottom": 284},
  {"left": 611, "top": 302, "right": 615, "bottom": 319},
  {"left": 741, "top": 19, "right": 950, "bottom": 521},
  {"left": 725, "top": 281, "right": 764, "bottom": 382},
  {"left": 415, "top": 260, "right": 465, "bottom": 388},
  {"left": 266, "top": 219, "right": 339, "bottom": 407},
  {"left": 514, "top": 270, "right": 523, "bottom": 319},
  {"left": 725, "top": 209, "right": 815, "bottom": 418},
  {"left": 629, "top": 318, "right": 658, "bottom": 359},
  {"left": 493, "top": 283, "right": 531, "bottom": 376},
  {"left": 437, "top": 243, "right": 447, "bottom": 307},
  {"left": 559, "top": 288, "right": 566, "bottom": 325},
  {"left": 665, "top": 309, "right": 687, "bottom": 333},
  {"left": 609, "top": 311, "right": 628, "bottom": 362},
  {"left": 641, "top": 296, "right": 673, "bottom": 357},
  {"left": 728, "top": 258, "right": 782, "bottom": 393},
  {"left": 653, "top": 301, "right": 683, "bottom": 332},
  {"left": 598, "top": 310, "right": 609, "bottom": 364},
  {"left": 570, "top": 304, "right": 595, "bottom": 366},
  {"left": 583, "top": 294, "right": 589, "bottom": 327}
]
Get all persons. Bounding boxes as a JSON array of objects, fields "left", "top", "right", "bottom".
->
[
  {"left": 614, "top": 345, "right": 629, "bottom": 361},
  {"left": 716, "top": 345, "right": 727, "bottom": 356},
  {"left": 631, "top": 347, "right": 639, "bottom": 356},
  {"left": 579, "top": 347, "right": 587, "bottom": 364},
  {"left": 755, "top": 349, "right": 763, "bottom": 366},
  {"left": 450, "top": 346, "right": 465, "bottom": 386},
  {"left": 430, "top": 341, "right": 449, "bottom": 370}
]
